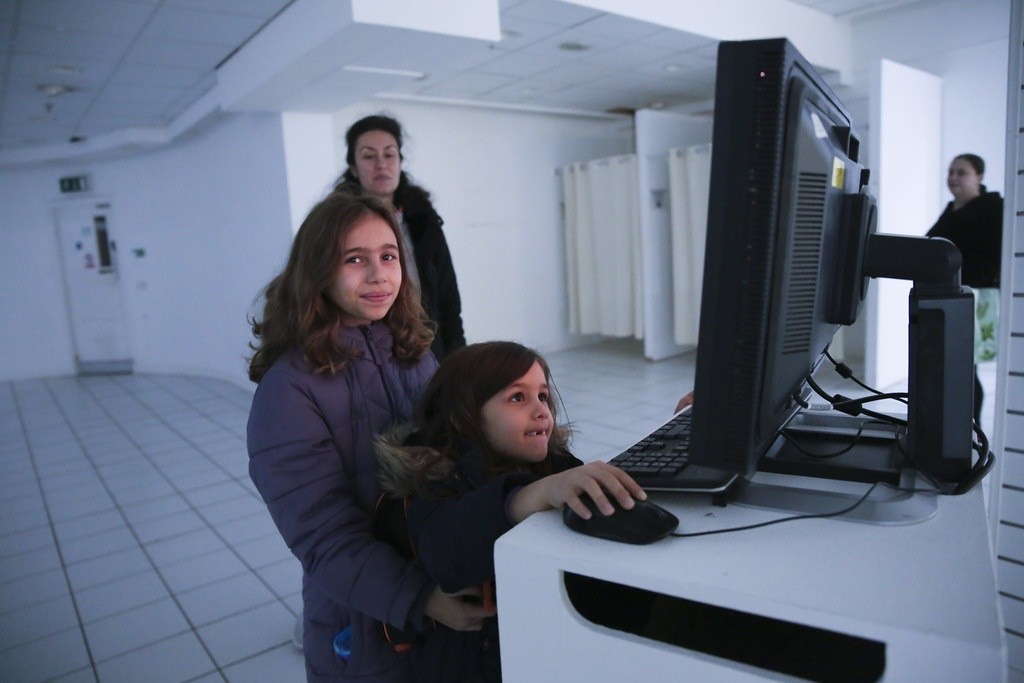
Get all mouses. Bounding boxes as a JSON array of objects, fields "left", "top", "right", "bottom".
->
[{"left": 562, "top": 490, "right": 681, "bottom": 544}]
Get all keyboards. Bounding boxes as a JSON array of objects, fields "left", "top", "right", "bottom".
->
[{"left": 608, "top": 387, "right": 812, "bottom": 493}]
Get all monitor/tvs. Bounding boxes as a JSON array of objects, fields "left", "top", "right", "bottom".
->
[{"left": 689, "top": 36, "right": 976, "bottom": 489}]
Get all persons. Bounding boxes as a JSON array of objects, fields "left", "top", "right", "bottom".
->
[
  {"left": 333, "top": 115, "right": 465, "bottom": 362},
  {"left": 245, "top": 186, "right": 694, "bottom": 683},
  {"left": 927, "top": 154, "right": 1004, "bottom": 362}
]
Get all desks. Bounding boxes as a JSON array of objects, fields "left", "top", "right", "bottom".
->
[{"left": 491, "top": 386, "right": 1012, "bottom": 683}]
